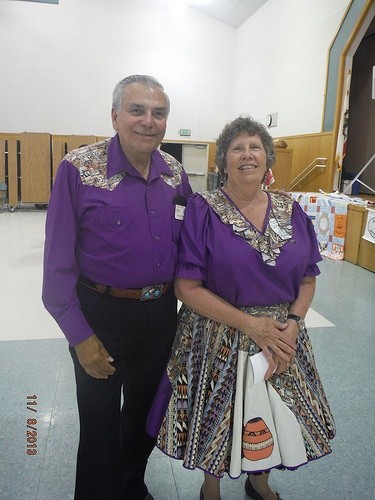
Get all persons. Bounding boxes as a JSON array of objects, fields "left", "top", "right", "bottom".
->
[
  {"left": 172, "top": 117, "right": 323, "bottom": 500},
  {"left": 41, "top": 74, "right": 291, "bottom": 500}
]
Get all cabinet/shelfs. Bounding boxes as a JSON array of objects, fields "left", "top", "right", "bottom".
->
[{"left": 263, "top": 148, "right": 292, "bottom": 193}]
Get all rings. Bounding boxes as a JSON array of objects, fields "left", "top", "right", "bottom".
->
[{"left": 285, "top": 364, "right": 289, "bottom": 365}]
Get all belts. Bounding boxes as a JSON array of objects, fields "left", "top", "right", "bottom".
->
[{"left": 77, "top": 274, "right": 174, "bottom": 302}]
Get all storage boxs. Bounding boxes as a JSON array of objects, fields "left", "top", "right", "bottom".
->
[{"left": 344, "top": 204, "right": 375, "bottom": 274}]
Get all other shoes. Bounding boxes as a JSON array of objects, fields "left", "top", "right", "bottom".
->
[
  {"left": 200, "top": 484, "right": 221, "bottom": 500},
  {"left": 245, "top": 475, "right": 283, "bottom": 500}
]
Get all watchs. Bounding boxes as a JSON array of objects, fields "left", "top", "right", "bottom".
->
[{"left": 287, "top": 314, "right": 305, "bottom": 330}]
